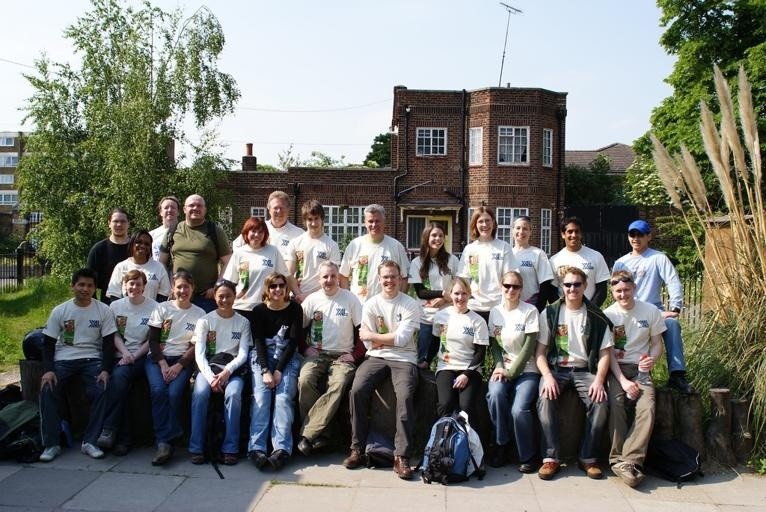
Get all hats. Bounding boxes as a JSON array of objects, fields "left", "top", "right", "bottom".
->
[{"left": 627, "top": 219, "right": 651, "bottom": 235}]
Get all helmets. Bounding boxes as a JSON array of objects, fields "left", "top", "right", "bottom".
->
[{"left": 21, "top": 328, "right": 44, "bottom": 361}]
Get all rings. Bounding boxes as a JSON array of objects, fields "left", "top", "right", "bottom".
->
[{"left": 173, "top": 375, "right": 175, "bottom": 377}]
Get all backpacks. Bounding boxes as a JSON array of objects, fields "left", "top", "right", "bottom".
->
[
  {"left": 421, "top": 413, "right": 468, "bottom": 485},
  {"left": 653, "top": 437, "right": 702, "bottom": 483},
  {"left": 203, "top": 410, "right": 226, "bottom": 466}
]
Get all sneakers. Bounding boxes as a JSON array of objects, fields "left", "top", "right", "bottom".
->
[
  {"left": 225, "top": 436, "right": 363, "bottom": 473},
  {"left": 392, "top": 456, "right": 412, "bottom": 480},
  {"left": 40, "top": 426, "right": 202, "bottom": 466},
  {"left": 491, "top": 450, "right": 648, "bottom": 488}
]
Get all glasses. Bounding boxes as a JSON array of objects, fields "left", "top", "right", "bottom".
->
[
  {"left": 380, "top": 274, "right": 399, "bottom": 280},
  {"left": 501, "top": 283, "right": 522, "bottom": 291},
  {"left": 629, "top": 232, "right": 644, "bottom": 238},
  {"left": 560, "top": 281, "right": 584, "bottom": 288},
  {"left": 268, "top": 284, "right": 286, "bottom": 289},
  {"left": 268, "top": 205, "right": 289, "bottom": 212},
  {"left": 214, "top": 280, "right": 234, "bottom": 287},
  {"left": 609, "top": 278, "right": 631, "bottom": 286}
]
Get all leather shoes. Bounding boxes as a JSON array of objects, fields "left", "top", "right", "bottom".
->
[{"left": 667, "top": 374, "right": 695, "bottom": 395}]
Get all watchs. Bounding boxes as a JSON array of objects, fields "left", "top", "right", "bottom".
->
[
  {"left": 671, "top": 307, "right": 680, "bottom": 313},
  {"left": 260, "top": 368, "right": 269, "bottom": 375}
]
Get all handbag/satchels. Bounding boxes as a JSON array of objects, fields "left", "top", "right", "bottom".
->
[
  {"left": 1, "top": 426, "right": 41, "bottom": 463},
  {"left": 364, "top": 429, "right": 393, "bottom": 469},
  {"left": 0, "top": 399, "right": 39, "bottom": 449}
]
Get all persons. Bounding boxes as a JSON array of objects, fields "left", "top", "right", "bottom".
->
[
  {"left": 95, "top": 269, "right": 159, "bottom": 456},
  {"left": 187, "top": 280, "right": 254, "bottom": 465},
  {"left": 602, "top": 271, "right": 667, "bottom": 488},
  {"left": 144, "top": 272, "right": 207, "bottom": 466},
  {"left": 511, "top": 216, "right": 556, "bottom": 315},
  {"left": 222, "top": 217, "right": 305, "bottom": 315},
  {"left": 297, "top": 260, "right": 367, "bottom": 455},
  {"left": 159, "top": 194, "right": 232, "bottom": 313},
  {"left": 406, "top": 222, "right": 460, "bottom": 370},
  {"left": 104, "top": 230, "right": 172, "bottom": 304},
  {"left": 39, "top": 268, "right": 119, "bottom": 463},
  {"left": 547, "top": 217, "right": 612, "bottom": 310},
  {"left": 611, "top": 220, "right": 695, "bottom": 395},
  {"left": 232, "top": 190, "right": 303, "bottom": 273},
  {"left": 246, "top": 272, "right": 304, "bottom": 471},
  {"left": 455, "top": 207, "right": 520, "bottom": 321},
  {"left": 425, "top": 277, "right": 490, "bottom": 431},
  {"left": 343, "top": 260, "right": 421, "bottom": 479},
  {"left": 338, "top": 204, "right": 410, "bottom": 304},
  {"left": 535, "top": 267, "right": 616, "bottom": 480},
  {"left": 147, "top": 197, "right": 180, "bottom": 264},
  {"left": 282, "top": 200, "right": 341, "bottom": 305},
  {"left": 485, "top": 271, "right": 543, "bottom": 474},
  {"left": 85, "top": 207, "right": 131, "bottom": 306}
]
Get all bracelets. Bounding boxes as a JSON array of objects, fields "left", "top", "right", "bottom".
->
[{"left": 123, "top": 351, "right": 129, "bottom": 356}]
